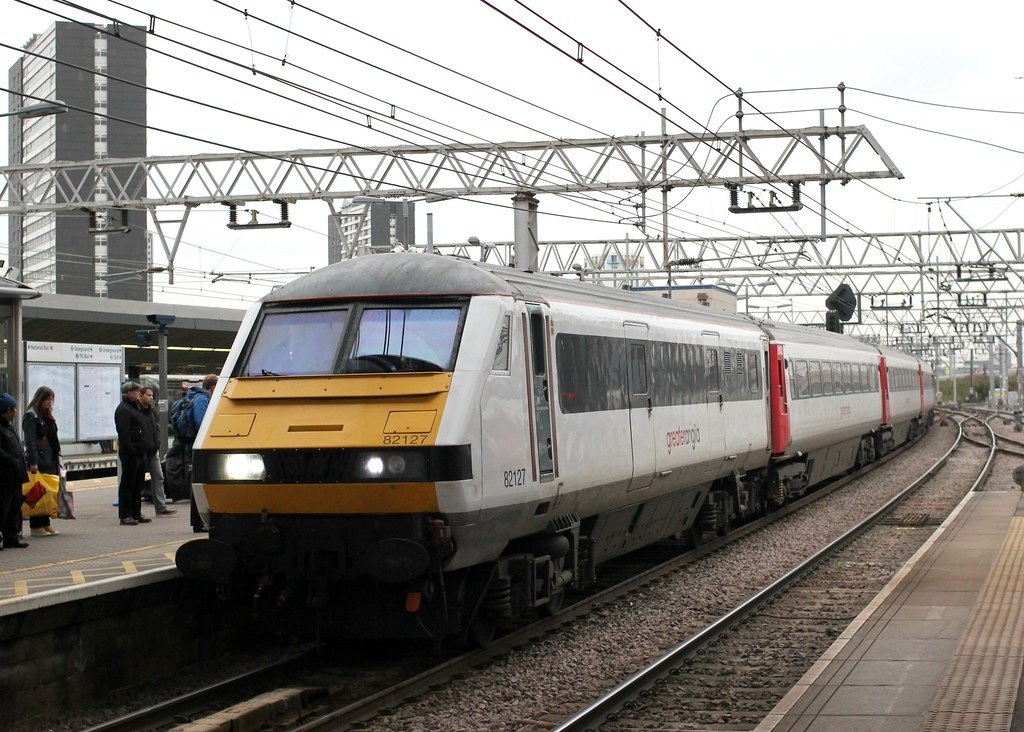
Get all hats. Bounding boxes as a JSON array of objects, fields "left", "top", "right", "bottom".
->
[
  {"left": 0, "top": 393, "right": 17, "bottom": 414},
  {"left": 120, "top": 381, "right": 144, "bottom": 394}
]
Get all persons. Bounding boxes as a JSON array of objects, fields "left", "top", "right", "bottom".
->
[
  {"left": 183, "top": 373, "right": 220, "bottom": 533},
  {"left": 136, "top": 386, "right": 178, "bottom": 518},
  {"left": 114, "top": 381, "right": 151, "bottom": 525},
  {"left": 21, "top": 387, "right": 64, "bottom": 536},
  {"left": 0, "top": 392, "right": 29, "bottom": 551}
]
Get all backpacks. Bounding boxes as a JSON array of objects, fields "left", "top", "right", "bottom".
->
[{"left": 171, "top": 392, "right": 207, "bottom": 443}]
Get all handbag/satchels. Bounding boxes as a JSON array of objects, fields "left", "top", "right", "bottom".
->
[
  {"left": 21, "top": 470, "right": 60, "bottom": 519},
  {"left": 57, "top": 470, "right": 76, "bottom": 519}
]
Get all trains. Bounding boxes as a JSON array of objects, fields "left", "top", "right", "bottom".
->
[{"left": 191, "top": 256, "right": 937, "bottom": 647}]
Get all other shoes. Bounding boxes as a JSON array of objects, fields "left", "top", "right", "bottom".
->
[
  {"left": 156, "top": 510, "right": 178, "bottom": 517},
  {"left": 30, "top": 527, "right": 60, "bottom": 536},
  {"left": 120, "top": 517, "right": 139, "bottom": 525},
  {"left": 134, "top": 516, "right": 152, "bottom": 523},
  {"left": 3, "top": 542, "right": 30, "bottom": 549}
]
